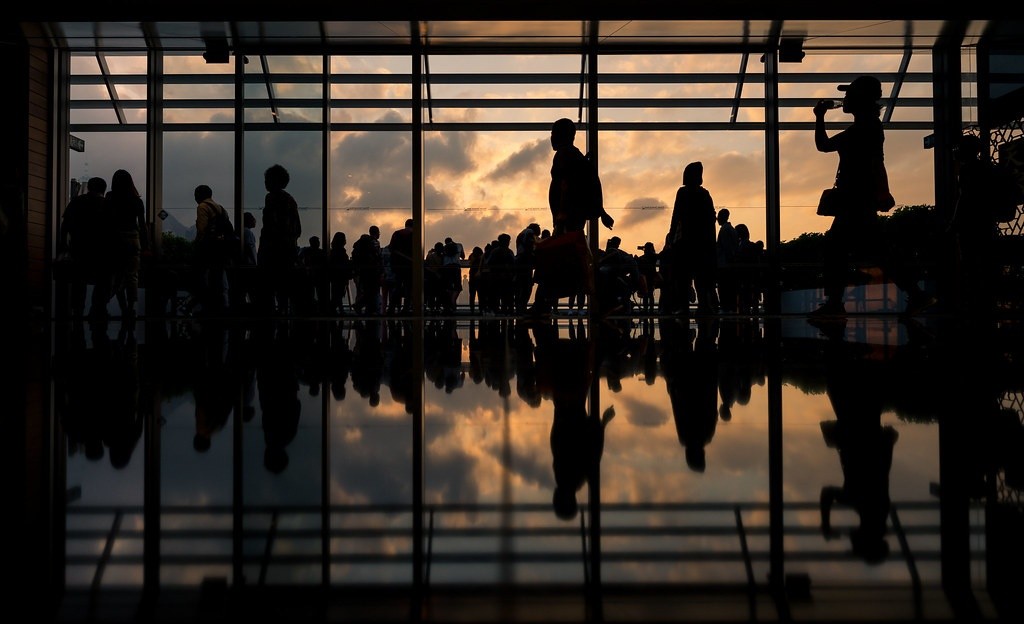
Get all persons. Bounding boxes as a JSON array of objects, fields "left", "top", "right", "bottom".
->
[
  {"left": 521, "top": 120, "right": 614, "bottom": 317},
  {"left": 242, "top": 213, "right": 673, "bottom": 317},
  {"left": 41, "top": 317, "right": 899, "bottom": 565},
  {"left": 191, "top": 185, "right": 231, "bottom": 317},
  {"left": 660, "top": 162, "right": 716, "bottom": 311},
  {"left": 62, "top": 177, "right": 115, "bottom": 318},
  {"left": 808, "top": 76, "right": 895, "bottom": 315},
  {"left": 714, "top": 209, "right": 760, "bottom": 315},
  {"left": 248, "top": 165, "right": 315, "bottom": 315},
  {"left": 104, "top": 170, "right": 144, "bottom": 318}
]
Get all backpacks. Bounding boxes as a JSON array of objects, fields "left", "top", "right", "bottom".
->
[
  {"left": 204, "top": 201, "right": 234, "bottom": 248},
  {"left": 444, "top": 242, "right": 458, "bottom": 256},
  {"left": 580, "top": 152, "right": 603, "bottom": 220}
]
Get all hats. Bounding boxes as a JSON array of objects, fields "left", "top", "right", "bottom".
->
[{"left": 837, "top": 76, "right": 881, "bottom": 92}]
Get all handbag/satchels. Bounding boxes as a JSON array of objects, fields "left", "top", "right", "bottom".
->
[
  {"left": 535, "top": 222, "right": 595, "bottom": 294},
  {"left": 817, "top": 189, "right": 838, "bottom": 217}
]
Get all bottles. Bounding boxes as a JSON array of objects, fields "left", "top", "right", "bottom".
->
[{"left": 818, "top": 99, "right": 844, "bottom": 109}]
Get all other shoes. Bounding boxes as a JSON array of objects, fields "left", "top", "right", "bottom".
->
[
  {"left": 578, "top": 310, "right": 585, "bottom": 315},
  {"left": 568, "top": 310, "right": 573, "bottom": 315},
  {"left": 899, "top": 292, "right": 939, "bottom": 318},
  {"left": 552, "top": 310, "right": 560, "bottom": 316},
  {"left": 807, "top": 300, "right": 846, "bottom": 319}
]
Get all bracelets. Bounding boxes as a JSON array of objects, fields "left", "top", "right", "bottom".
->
[{"left": 599, "top": 207, "right": 603, "bottom": 212}]
